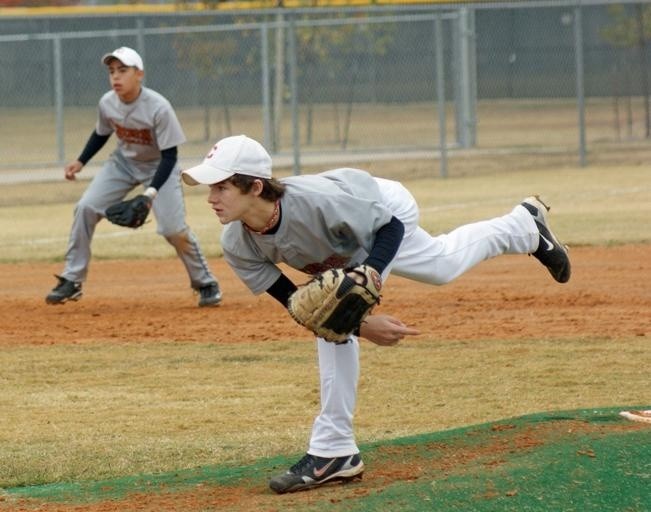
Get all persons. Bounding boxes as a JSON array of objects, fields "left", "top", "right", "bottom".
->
[
  {"left": 43, "top": 44, "right": 222, "bottom": 308},
  {"left": 180, "top": 135, "right": 572, "bottom": 496}
]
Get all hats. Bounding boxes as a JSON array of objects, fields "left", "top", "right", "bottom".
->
[
  {"left": 183, "top": 136, "right": 271, "bottom": 185},
  {"left": 104, "top": 47, "right": 144, "bottom": 71}
]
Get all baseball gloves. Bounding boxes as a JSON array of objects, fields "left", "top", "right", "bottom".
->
[
  {"left": 105, "top": 193, "right": 152, "bottom": 228},
  {"left": 287, "top": 263, "right": 381, "bottom": 345}
]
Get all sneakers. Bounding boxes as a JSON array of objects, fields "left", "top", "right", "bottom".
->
[
  {"left": 270, "top": 454, "right": 364, "bottom": 492},
  {"left": 199, "top": 282, "right": 222, "bottom": 305},
  {"left": 46, "top": 275, "right": 83, "bottom": 302},
  {"left": 521, "top": 196, "right": 570, "bottom": 282}
]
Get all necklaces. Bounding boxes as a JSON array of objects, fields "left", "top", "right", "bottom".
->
[{"left": 242, "top": 198, "right": 279, "bottom": 235}]
